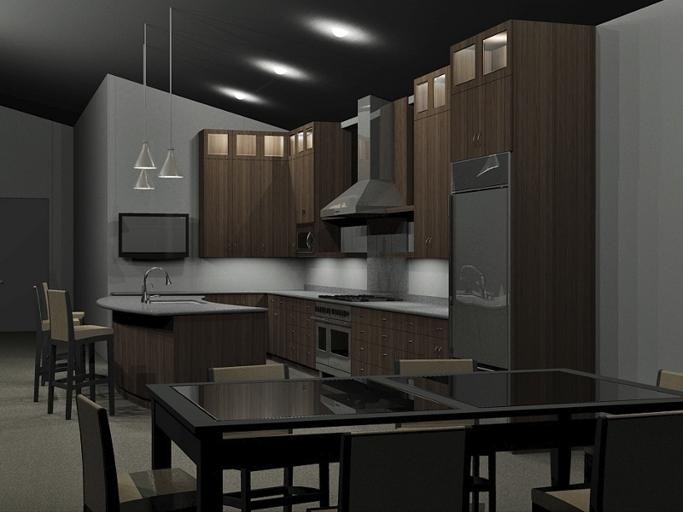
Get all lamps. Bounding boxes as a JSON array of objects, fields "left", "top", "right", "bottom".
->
[
  {"left": 134, "top": 23, "right": 156, "bottom": 170},
  {"left": 159, "top": 8, "right": 181, "bottom": 178},
  {"left": 132, "top": 170, "right": 155, "bottom": 191}
]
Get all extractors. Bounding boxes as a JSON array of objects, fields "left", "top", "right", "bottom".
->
[{"left": 321, "top": 95, "right": 415, "bottom": 221}]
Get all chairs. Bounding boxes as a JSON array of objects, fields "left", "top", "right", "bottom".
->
[
  {"left": 75, "top": 393, "right": 197, "bottom": 512},
  {"left": 306, "top": 425, "right": 474, "bottom": 512},
  {"left": 530, "top": 409, "right": 682, "bottom": 512},
  {"left": 394, "top": 358, "right": 496, "bottom": 512},
  {"left": 209, "top": 361, "right": 329, "bottom": 512},
  {"left": 39, "top": 278, "right": 86, "bottom": 382},
  {"left": 31, "top": 280, "right": 81, "bottom": 402},
  {"left": 46, "top": 288, "right": 115, "bottom": 420},
  {"left": 657, "top": 369, "right": 683, "bottom": 392}
]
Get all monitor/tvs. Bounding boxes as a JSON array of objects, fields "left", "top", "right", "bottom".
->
[{"left": 118, "top": 212, "right": 189, "bottom": 262}]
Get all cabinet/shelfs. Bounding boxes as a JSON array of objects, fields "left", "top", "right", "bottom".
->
[
  {"left": 449, "top": 19, "right": 594, "bottom": 371},
  {"left": 413, "top": 65, "right": 450, "bottom": 259},
  {"left": 267, "top": 294, "right": 315, "bottom": 369},
  {"left": 350, "top": 306, "right": 448, "bottom": 377},
  {"left": 289, "top": 122, "right": 352, "bottom": 259},
  {"left": 198, "top": 129, "right": 289, "bottom": 258}
]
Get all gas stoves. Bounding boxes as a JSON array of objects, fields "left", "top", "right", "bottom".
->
[{"left": 319, "top": 295, "right": 403, "bottom": 302}]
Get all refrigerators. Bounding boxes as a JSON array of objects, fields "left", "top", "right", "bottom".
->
[{"left": 447, "top": 153, "right": 510, "bottom": 371}]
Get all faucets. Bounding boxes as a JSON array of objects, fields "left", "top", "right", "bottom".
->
[{"left": 140, "top": 266, "right": 173, "bottom": 303}]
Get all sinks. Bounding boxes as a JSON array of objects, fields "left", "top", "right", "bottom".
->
[{"left": 154, "top": 298, "right": 207, "bottom": 306}]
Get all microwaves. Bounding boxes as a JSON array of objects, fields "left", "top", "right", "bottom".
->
[{"left": 295, "top": 226, "right": 314, "bottom": 254}]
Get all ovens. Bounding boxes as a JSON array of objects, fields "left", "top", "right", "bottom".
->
[{"left": 311, "top": 317, "right": 352, "bottom": 373}]
